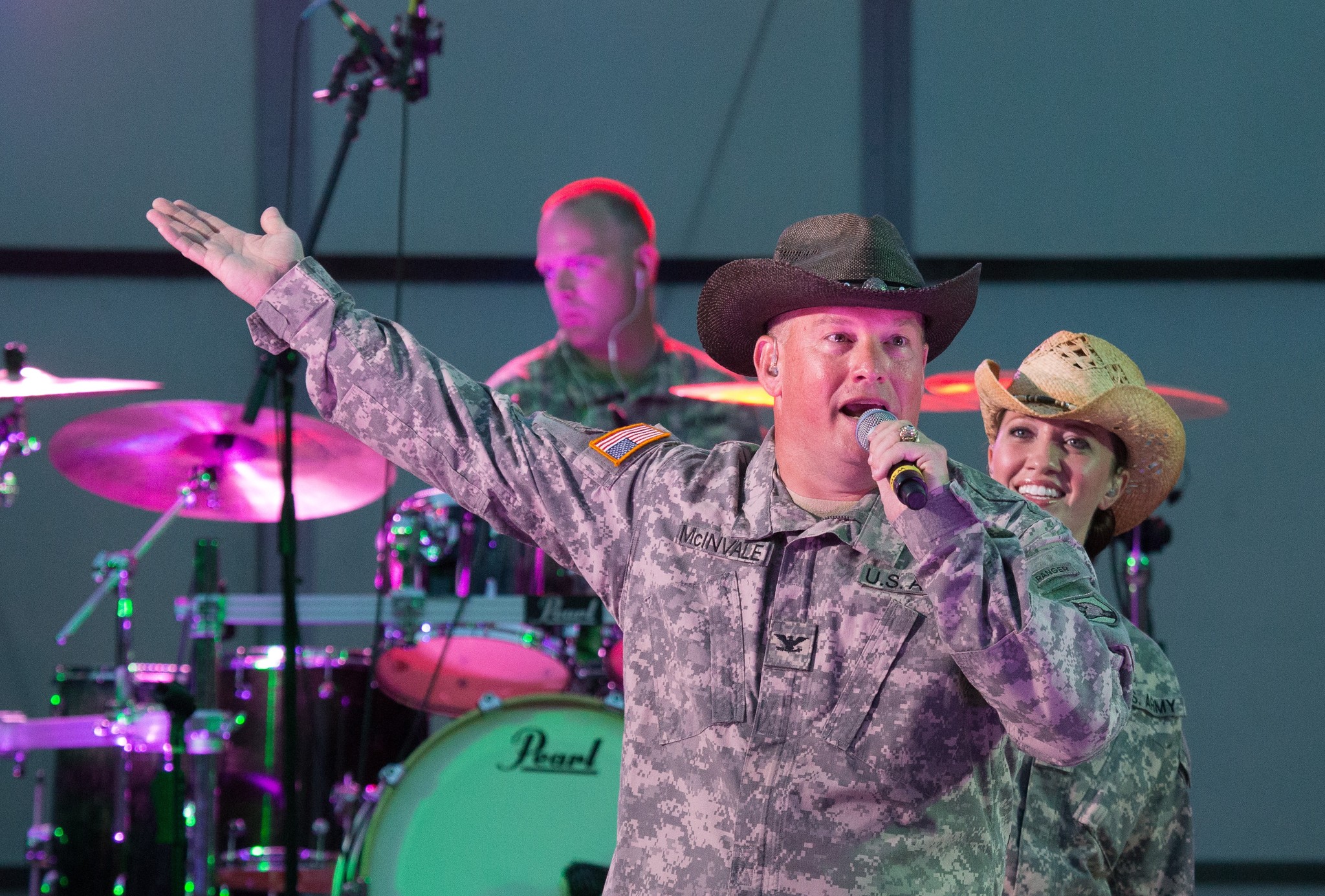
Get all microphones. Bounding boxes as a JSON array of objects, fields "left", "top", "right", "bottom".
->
[
  {"left": 856, "top": 409, "right": 927, "bottom": 510},
  {"left": 324, "top": 0, "right": 418, "bottom": 98}
]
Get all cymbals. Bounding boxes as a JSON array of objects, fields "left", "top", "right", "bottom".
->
[
  {"left": 666, "top": 368, "right": 985, "bottom": 427},
  {"left": 0, "top": 365, "right": 163, "bottom": 401},
  {"left": 48, "top": 398, "right": 397, "bottom": 524},
  {"left": 922, "top": 367, "right": 1229, "bottom": 421}
]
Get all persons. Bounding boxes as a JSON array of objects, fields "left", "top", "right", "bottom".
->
[
  {"left": 481, "top": 176, "right": 766, "bottom": 460},
  {"left": 972, "top": 327, "right": 1199, "bottom": 896},
  {"left": 142, "top": 194, "right": 1137, "bottom": 896}
]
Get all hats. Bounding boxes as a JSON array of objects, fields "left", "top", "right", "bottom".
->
[
  {"left": 697, "top": 211, "right": 983, "bottom": 376},
  {"left": 974, "top": 329, "right": 1185, "bottom": 539}
]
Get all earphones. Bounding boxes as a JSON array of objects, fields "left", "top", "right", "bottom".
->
[{"left": 634, "top": 267, "right": 645, "bottom": 289}]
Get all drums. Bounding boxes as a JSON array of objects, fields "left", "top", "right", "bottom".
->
[
  {"left": 329, "top": 692, "right": 625, "bottom": 895},
  {"left": 373, "top": 488, "right": 583, "bottom": 717},
  {"left": 214, "top": 641, "right": 397, "bottom": 889},
  {"left": 53, "top": 661, "right": 194, "bottom": 896}
]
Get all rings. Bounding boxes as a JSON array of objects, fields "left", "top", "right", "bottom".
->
[{"left": 900, "top": 425, "right": 920, "bottom": 443}]
